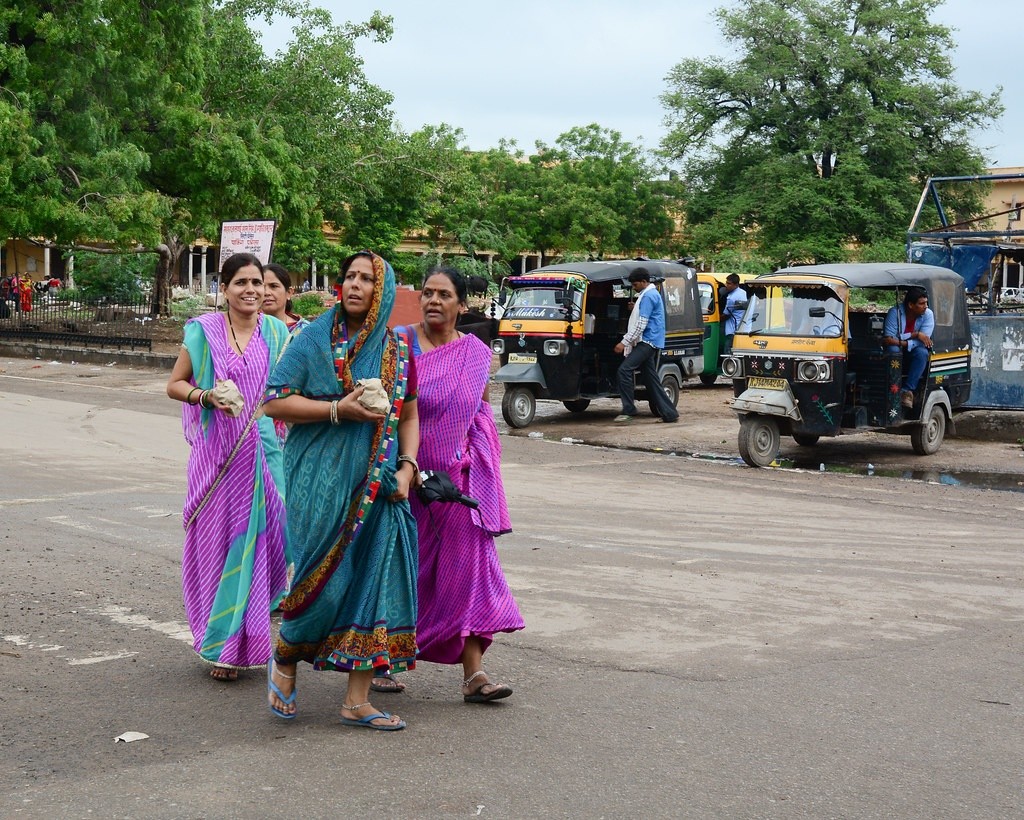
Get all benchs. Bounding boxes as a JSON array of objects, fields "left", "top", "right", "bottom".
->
[
  {"left": 585, "top": 316, "right": 629, "bottom": 349},
  {"left": 847, "top": 310, "right": 891, "bottom": 359}
]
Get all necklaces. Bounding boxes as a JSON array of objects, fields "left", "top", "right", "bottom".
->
[
  {"left": 227, "top": 309, "right": 243, "bottom": 354},
  {"left": 420, "top": 322, "right": 461, "bottom": 348}
]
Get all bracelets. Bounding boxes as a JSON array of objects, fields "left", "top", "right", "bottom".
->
[
  {"left": 330, "top": 400, "right": 342, "bottom": 426},
  {"left": 188, "top": 387, "right": 201, "bottom": 406},
  {"left": 198, "top": 390, "right": 214, "bottom": 410},
  {"left": 397, "top": 455, "right": 419, "bottom": 480}
]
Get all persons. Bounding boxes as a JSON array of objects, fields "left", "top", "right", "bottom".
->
[
  {"left": 168, "top": 253, "right": 289, "bottom": 681},
  {"left": 720, "top": 273, "right": 748, "bottom": 355},
  {"left": 263, "top": 264, "right": 310, "bottom": 449},
  {"left": 261, "top": 248, "right": 420, "bottom": 731},
  {"left": 210, "top": 276, "right": 217, "bottom": 293},
  {"left": 393, "top": 266, "right": 526, "bottom": 703},
  {"left": 614, "top": 267, "right": 678, "bottom": 424},
  {"left": 304, "top": 278, "right": 309, "bottom": 287},
  {"left": 3, "top": 272, "right": 38, "bottom": 315},
  {"left": 881, "top": 286, "right": 934, "bottom": 408}
]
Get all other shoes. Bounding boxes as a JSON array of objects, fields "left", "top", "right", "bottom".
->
[
  {"left": 654, "top": 417, "right": 679, "bottom": 424},
  {"left": 902, "top": 390, "right": 913, "bottom": 408},
  {"left": 614, "top": 415, "right": 633, "bottom": 422}
]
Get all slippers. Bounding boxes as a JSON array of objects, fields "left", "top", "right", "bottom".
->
[
  {"left": 341, "top": 707, "right": 407, "bottom": 731},
  {"left": 267, "top": 658, "right": 297, "bottom": 719},
  {"left": 461, "top": 671, "right": 512, "bottom": 702},
  {"left": 369, "top": 673, "right": 405, "bottom": 693},
  {"left": 211, "top": 667, "right": 239, "bottom": 682}
]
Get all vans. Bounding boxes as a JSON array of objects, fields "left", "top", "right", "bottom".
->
[{"left": 986, "top": 287, "right": 1024, "bottom": 304}]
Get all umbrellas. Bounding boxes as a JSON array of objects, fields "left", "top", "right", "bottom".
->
[{"left": 414, "top": 470, "right": 477, "bottom": 540}]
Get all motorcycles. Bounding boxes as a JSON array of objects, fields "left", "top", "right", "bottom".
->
[
  {"left": 490, "top": 260, "right": 705, "bottom": 430},
  {"left": 667, "top": 272, "right": 786, "bottom": 385},
  {"left": 720, "top": 263, "right": 973, "bottom": 467}
]
